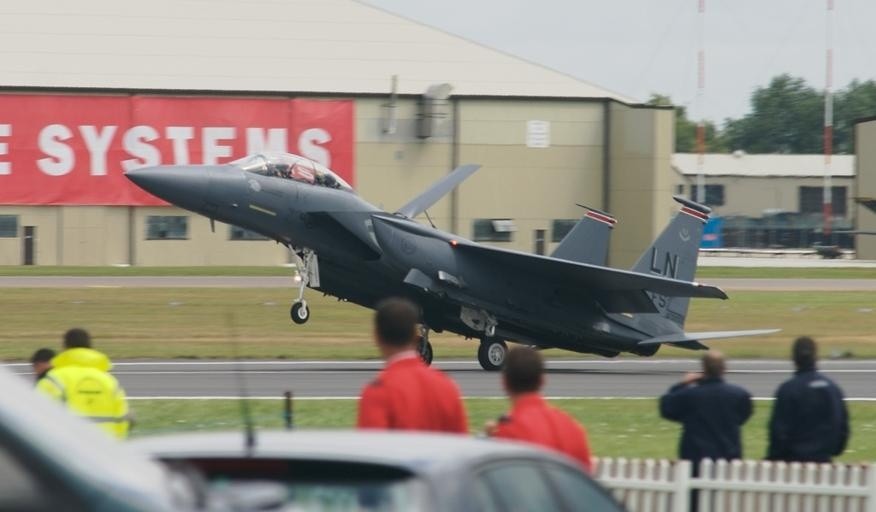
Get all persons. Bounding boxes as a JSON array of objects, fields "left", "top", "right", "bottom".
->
[
  {"left": 485, "top": 346, "right": 591, "bottom": 477},
  {"left": 661, "top": 348, "right": 754, "bottom": 510},
  {"left": 30, "top": 346, "right": 57, "bottom": 384},
  {"left": 355, "top": 297, "right": 469, "bottom": 444},
  {"left": 763, "top": 332, "right": 853, "bottom": 476},
  {"left": 34, "top": 325, "right": 131, "bottom": 444}
]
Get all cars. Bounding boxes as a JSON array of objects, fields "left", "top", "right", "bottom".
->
[
  {"left": 722, "top": 210, "right": 853, "bottom": 249},
  {"left": 120, "top": 424, "right": 625, "bottom": 512}
]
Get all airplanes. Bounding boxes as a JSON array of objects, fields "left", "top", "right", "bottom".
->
[{"left": 122, "top": 150, "right": 782, "bottom": 374}]
